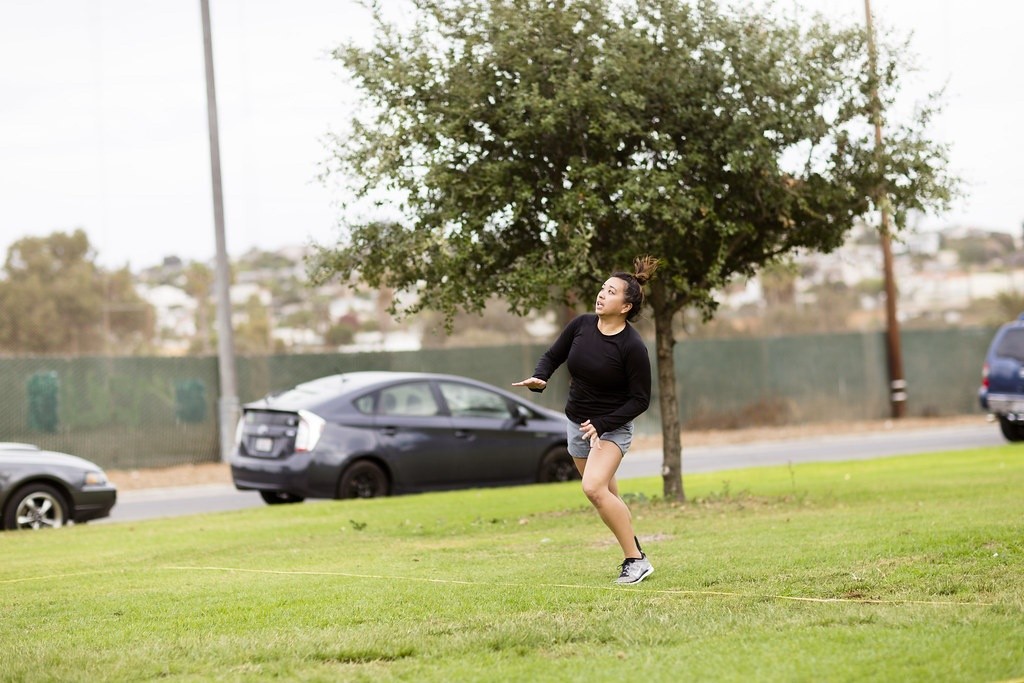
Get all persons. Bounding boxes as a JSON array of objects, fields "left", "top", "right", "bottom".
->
[{"left": 512, "top": 256, "right": 661, "bottom": 585}]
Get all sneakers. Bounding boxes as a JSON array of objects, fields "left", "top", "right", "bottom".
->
[{"left": 613, "top": 553, "right": 655, "bottom": 586}]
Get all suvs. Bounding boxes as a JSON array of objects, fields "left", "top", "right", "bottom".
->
[{"left": 976, "top": 313, "right": 1024, "bottom": 442}]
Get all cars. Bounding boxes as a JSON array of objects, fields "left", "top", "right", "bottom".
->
[
  {"left": 229, "top": 371, "right": 596, "bottom": 506},
  {"left": 0, "top": 441, "right": 117, "bottom": 534}
]
